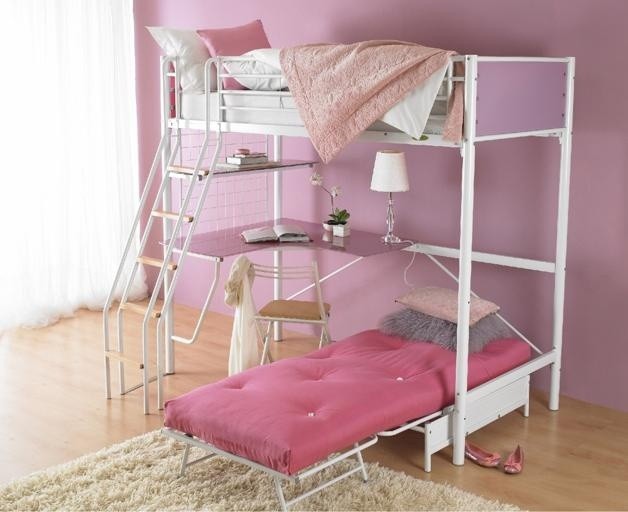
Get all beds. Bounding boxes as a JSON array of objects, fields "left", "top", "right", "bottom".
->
[{"left": 105, "top": 46, "right": 577, "bottom": 509}]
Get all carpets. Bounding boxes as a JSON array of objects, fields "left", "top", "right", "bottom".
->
[{"left": 2, "top": 420, "right": 526, "bottom": 509}]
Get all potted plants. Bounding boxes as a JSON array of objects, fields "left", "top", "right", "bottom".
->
[
  {"left": 321, "top": 219, "right": 337, "bottom": 232},
  {"left": 327, "top": 206, "right": 351, "bottom": 239}
]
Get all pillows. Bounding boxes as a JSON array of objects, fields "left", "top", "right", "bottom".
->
[
  {"left": 143, "top": 22, "right": 222, "bottom": 94},
  {"left": 378, "top": 306, "right": 513, "bottom": 356},
  {"left": 195, "top": 19, "right": 275, "bottom": 91},
  {"left": 394, "top": 285, "right": 502, "bottom": 330}
]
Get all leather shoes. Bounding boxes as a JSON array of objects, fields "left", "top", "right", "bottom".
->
[
  {"left": 465, "top": 440, "right": 501, "bottom": 468},
  {"left": 505, "top": 444, "right": 524, "bottom": 473}
]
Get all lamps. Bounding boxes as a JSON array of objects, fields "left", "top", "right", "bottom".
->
[{"left": 368, "top": 150, "right": 413, "bottom": 246}]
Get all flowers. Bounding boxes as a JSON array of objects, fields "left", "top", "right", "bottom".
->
[{"left": 308, "top": 172, "right": 342, "bottom": 223}]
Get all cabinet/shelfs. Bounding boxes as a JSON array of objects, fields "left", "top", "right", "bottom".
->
[{"left": 162, "top": 149, "right": 418, "bottom": 267}]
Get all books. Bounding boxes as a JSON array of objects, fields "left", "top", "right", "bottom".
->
[
  {"left": 239, "top": 223, "right": 310, "bottom": 243},
  {"left": 215, "top": 151, "right": 280, "bottom": 169}
]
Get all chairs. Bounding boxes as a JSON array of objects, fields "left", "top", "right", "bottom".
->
[{"left": 239, "top": 256, "right": 333, "bottom": 367}]
